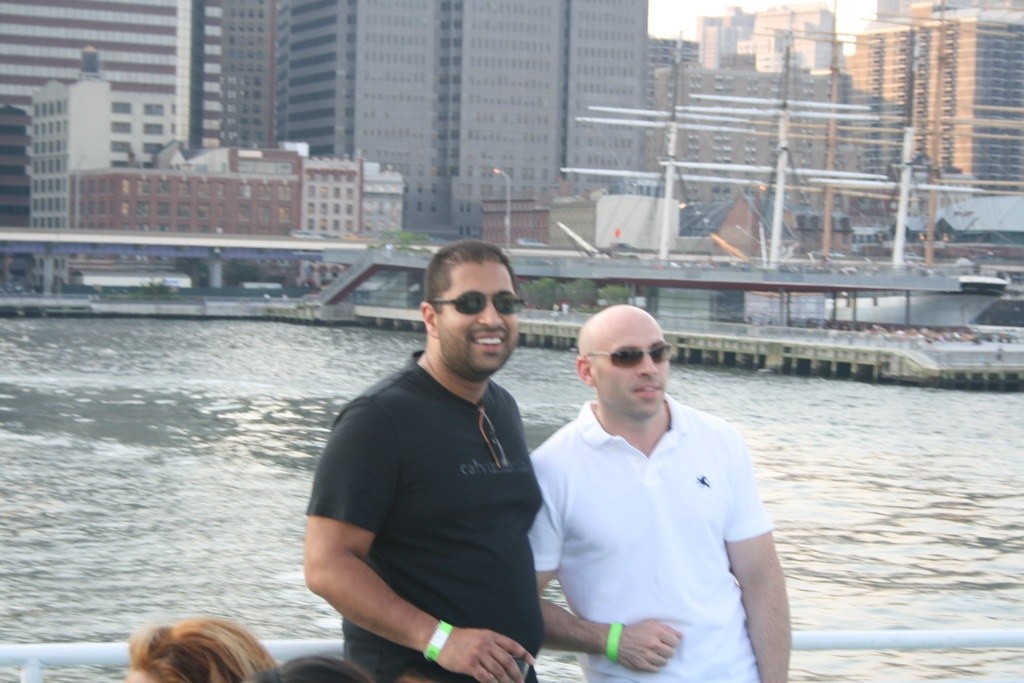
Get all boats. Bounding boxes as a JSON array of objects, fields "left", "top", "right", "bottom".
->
[{"left": 550, "top": 23, "right": 1024, "bottom": 335}]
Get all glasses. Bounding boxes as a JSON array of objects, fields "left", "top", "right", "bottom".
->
[
  {"left": 428, "top": 291, "right": 526, "bottom": 316},
  {"left": 478, "top": 407, "right": 508, "bottom": 468},
  {"left": 584, "top": 344, "right": 672, "bottom": 369}
]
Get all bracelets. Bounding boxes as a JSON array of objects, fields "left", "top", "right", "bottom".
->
[
  {"left": 607, "top": 622, "right": 623, "bottom": 660},
  {"left": 425, "top": 621, "right": 452, "bottom": 661}
]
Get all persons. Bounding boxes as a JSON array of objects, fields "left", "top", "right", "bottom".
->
[
  {"left": 529, "top": 304, "right": 793, "bottom": 683},
  {"left": 128, "top": 617, "right": 277, "bottom": 683},
  {"left": 304, "top": 241, "right": 545, "bottom": 683},
  {"left": 247, "top": 655, "right": 372, "bottom": 683}
]
